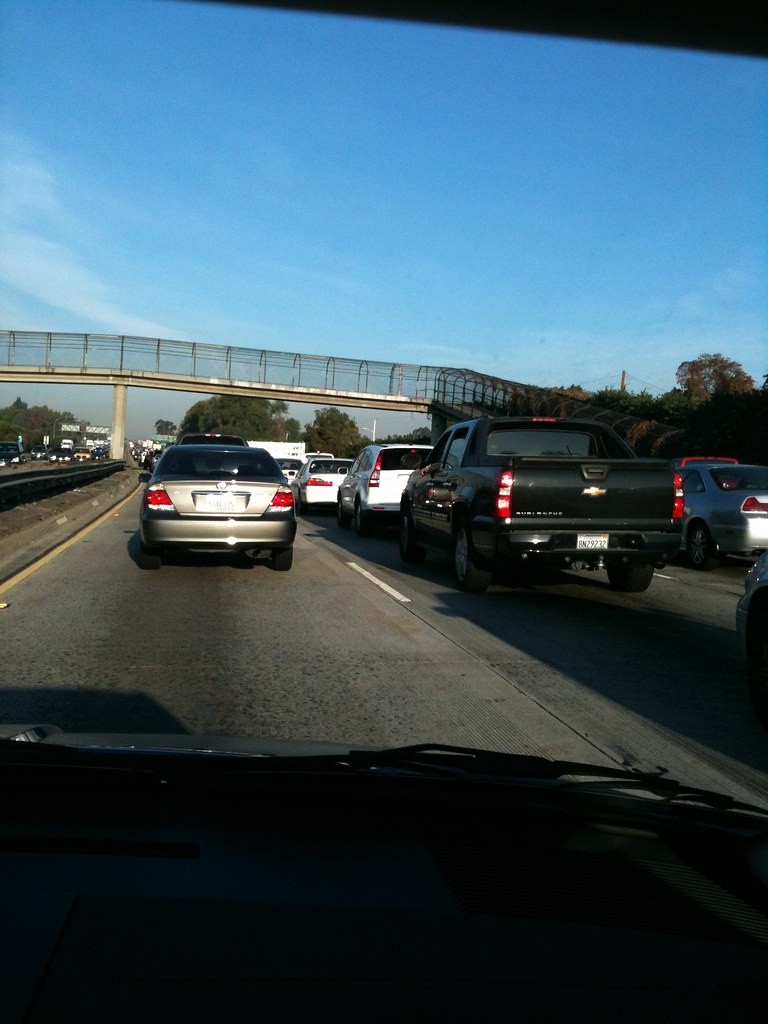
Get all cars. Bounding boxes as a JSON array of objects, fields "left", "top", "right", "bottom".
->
[
  {"left": 31, "top": 438, "right": 109, "bottom": 465},
  {"left": 0, "top": 441, "right": 27, "bottom": 467},
  {"left": 673, "top": 463, "right": 768, "bottom": 573},
  {"left": 735, "top": 547, "right": 768, "bottom": 725},
  {"left": 130, "top": 445, "right": 163, "bottom": 474},
  {"left": 291, "top": 458, "right": 356, "bottom": 515},
  {"left": 272, "top": 452, "right": 334, "bottom": 486},
  {"left": 138, "top": 442, "right": 297, "bottom": 570}
]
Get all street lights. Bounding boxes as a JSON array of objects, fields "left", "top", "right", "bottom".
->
[{"left": 53, "top": 415, "right": 72, "bottom": 440}]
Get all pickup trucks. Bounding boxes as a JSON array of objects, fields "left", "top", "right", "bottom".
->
[{"left": 399, "top": 414, "right": 685, "bottom": 592}]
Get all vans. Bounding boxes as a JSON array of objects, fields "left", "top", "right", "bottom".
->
[
  {"left": 178, "top": 433, "right": 250, "bottom": 447},
  {"left": 672, "top": 455, "right": 740, "bottom": 519}
]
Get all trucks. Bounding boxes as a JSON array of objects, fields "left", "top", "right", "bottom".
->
[{"left": 247, "top": 442, "right": 305, "bottom": 460}]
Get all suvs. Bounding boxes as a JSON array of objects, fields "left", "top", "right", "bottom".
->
[{"left": 337, "top": 442, "right": 437, "bottom": 537}]
